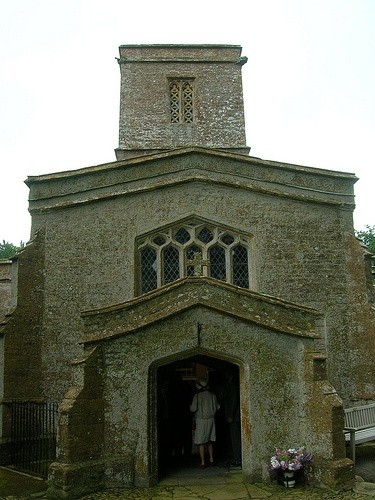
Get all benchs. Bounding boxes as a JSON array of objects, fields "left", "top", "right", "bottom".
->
[{"left": 344, "top": 404, "right": 375, "bottom": 465}]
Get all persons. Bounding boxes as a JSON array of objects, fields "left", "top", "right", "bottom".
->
[
  {"left": 167, "top": 419, "right": 184, "bottom": 457},
  {"left": 190, "top": 379, "right": 220, "bottom": 469},
  {"left": 221, "top": 368, "right": 242, "bottom": 466},
  {"left": 157, "top": 379, "right": 169, "bottom": 459}
]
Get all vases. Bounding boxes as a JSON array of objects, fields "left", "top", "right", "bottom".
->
[{"left": 284, "top": 471, "right": 295, "bottom": 488}]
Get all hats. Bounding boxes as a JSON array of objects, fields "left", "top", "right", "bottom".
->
[{"left": 196, "top": 380, "right": 209, "bottom": 390}]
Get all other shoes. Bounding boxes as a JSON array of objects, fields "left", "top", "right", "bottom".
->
[
  {"left": 200, "top": 463, "right": 207, "bottom": 469},
  {"left": 209, "top": 460, "right": 216, "bottom": 465}
]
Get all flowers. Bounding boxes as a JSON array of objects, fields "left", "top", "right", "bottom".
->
[{"left": 271, "top": 446, "right": 312, "bottom": 470}]
